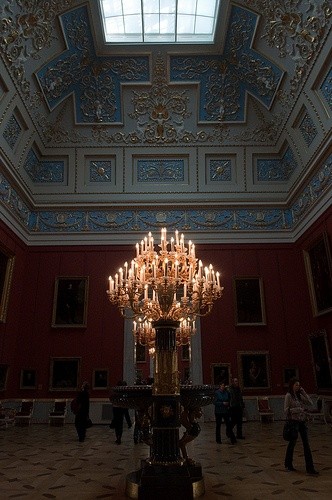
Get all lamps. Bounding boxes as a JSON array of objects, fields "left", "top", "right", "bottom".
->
[{"left": 107, "top": 225, "right": 223, "bottom": 355}]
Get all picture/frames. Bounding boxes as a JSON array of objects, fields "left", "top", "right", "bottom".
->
[
  {"left": 50, "top": 274, "right": 90, "bottom": 329},
  {"left": 0, "top": 362, "right": 11, "bottom": 392},
  {"left": 0, "top": 241, "right": 16, "bottom": 324},
  {"left": 48, "top": 356, "right": 81, "bottom": 392},
  {"left": 281, "top": 367, "right": 300, "bottom": 388},
  {"left": 236, "top": 350, "right": 272, "bottom": 389},
  {"left": 302, "top": 231, "right": 332, "bottom": 317},
  {"left": 305, "top": 329, "right": 332, "bottom": 391},
  {"left": 19, "top": 367, "right": 40, "bottom": 390},
  {"left": 231, "top": 271, "right": 268, "bottom": 327},
  {"left": 208, "top": 362, "right": 233, "bottom": 387},
  {"left": 91, "top": 367, "right": 110, "bottom": 390}
]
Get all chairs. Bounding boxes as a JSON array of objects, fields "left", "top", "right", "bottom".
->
[
  {"left": 309, "top": 396, "right": 328, "bottom": 426},
  {"left": 48, "top": 398, "right": 67, "bottom": 426},
  {"left": 15, "top": 398, "right": 35, "bottom": 427},
  {"left": 256, "top": 395, "right": 277, "bottom": 422},
  {"left": 0, "top": 401, "right": 15, "bottom": 430}
]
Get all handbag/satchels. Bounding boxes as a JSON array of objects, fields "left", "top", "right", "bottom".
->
[
  {"left": 110, "top": 418, "right": 115, "bottom": 429},
  {"left": 84, "top": 417, "right": 93, "bottom": 429},
  {"left": 283, "top": 412, "right": 298, "bottom": 441}
]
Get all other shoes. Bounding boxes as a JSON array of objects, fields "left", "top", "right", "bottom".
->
[
  {"left": 306, "top": 470, "right": 320, "bottom": 475},
  {"left": 236, "top": 436, "right": 245, "bottom": 439},
  {"left": 284, "top": 463, "right": 296, "bottom": 471},
  {"left": 115, "top": 439, "right": 121, "bottom": 445}
]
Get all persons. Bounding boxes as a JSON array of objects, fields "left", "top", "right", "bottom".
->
[
  {"left": 110, "top": 381, "right": 132, "bottom": 444},
  {"left": 71, "top": 383, "right": 89, "bottom": 442},
  {"left": 289, "top": 24, "right": 317, "bottom": 44},
  {"left": 149, "top": 113, "right": 168, "bottom": 138},
  {"left": 284, "top": 377, "right": 320, "bottom": 473},
  {"left": 226, "top": 378, "right": 246, "bottom": 440},
  {"left": 47, "top": 77, "right": 62, "bottom": 91},
  {"left": 218, "top": 99, "right": 230, "bottom": 118},
  {"left": 257, "top": 76, "right": 272, "bottom": 90},
  {"left": 213, "top": 381, "right": 240, "bottom": 441},
  {"left": 95, "top": 100, "right": 102, "bottom": 121}
]
